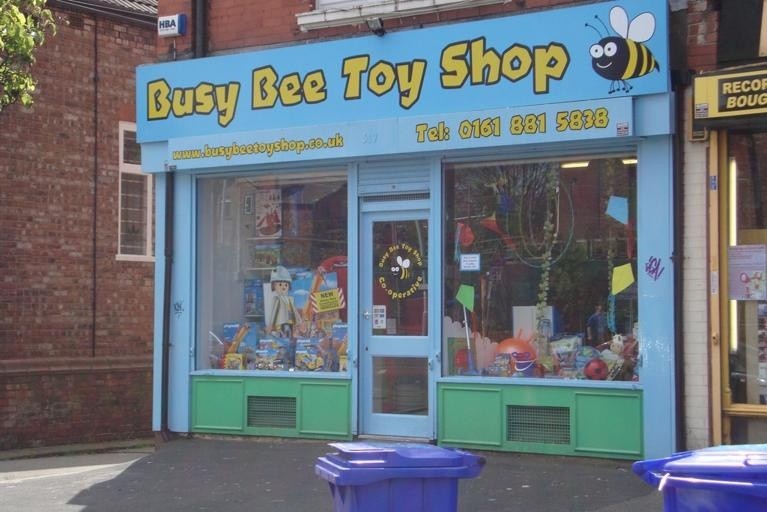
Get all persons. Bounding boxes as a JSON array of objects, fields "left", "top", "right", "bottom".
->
[{"left": 585, "top": 302, "right": 613, "bottom": 349}]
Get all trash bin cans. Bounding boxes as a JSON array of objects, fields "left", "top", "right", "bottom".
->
[
  {"left": 631, "top": 443, "right": 767, "bottom": 512},
  {"left": 314, "top": 441, "right": 487, "bottom": 512}
]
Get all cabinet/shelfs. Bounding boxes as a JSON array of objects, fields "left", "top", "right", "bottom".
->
[{"left": 244, "top": 236, "right": 347, "bottom": 317}]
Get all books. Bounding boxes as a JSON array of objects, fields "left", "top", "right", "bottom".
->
[{"left": 446, "top": 336, "right": 474, "bottom": 376}]
